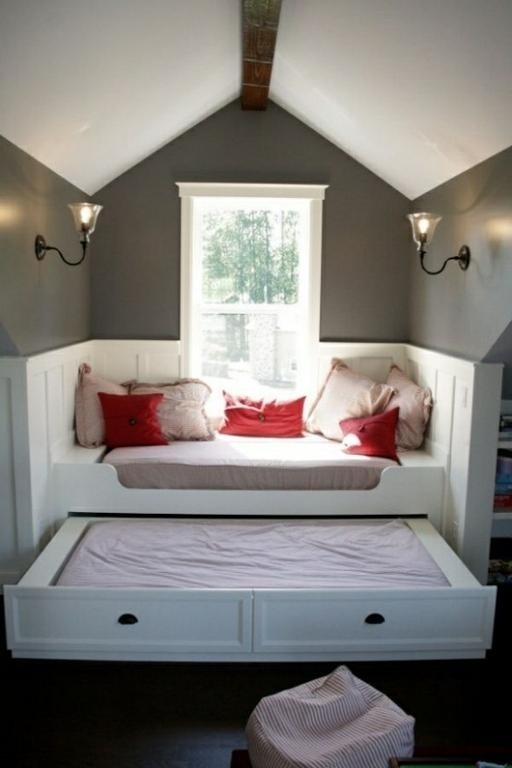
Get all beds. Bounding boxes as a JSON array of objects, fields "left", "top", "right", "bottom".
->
[{"left": 0, "top": 337, "right": 506, "bottom": 662}]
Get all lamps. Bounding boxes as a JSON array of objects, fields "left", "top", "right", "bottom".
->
[
  {"left": 34, "top": 202, "right": 105, "bottom": 266},
  {"left": 404, "top": 211, "right": 471, "bottom": 276}
]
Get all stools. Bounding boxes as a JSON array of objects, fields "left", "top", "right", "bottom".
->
[{"left": 249, "top": 672, "right": 414, "bottom": 768}]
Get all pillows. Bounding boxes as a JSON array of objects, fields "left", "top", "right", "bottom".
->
[
  {"left": 339, "top": 406, "right": 403, "bottom": 466},
  {"left": 97, "top": 391, "right": 169, "bottom": 447},
  {"left": 383, "top": 365, "right": 432, "bottom": 452},
  {"left": 305, "top": 361, "right": 395, "bottom": 443},
  {"left": 218, "top": 389, "right": 306, "bottom": 439},
  {"left": 76, "top": 362, "right": 215, "bottom": 448}
]
